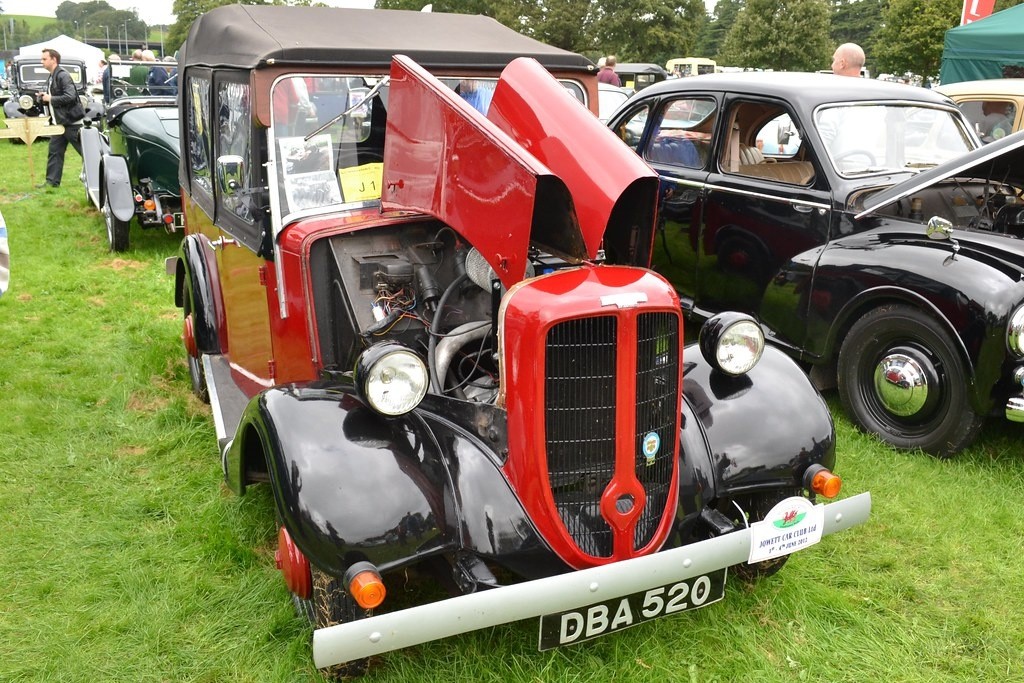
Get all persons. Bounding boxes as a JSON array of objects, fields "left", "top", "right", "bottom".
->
[
  {"left": 979, "top": 100, "right": 1014, "bottom": 141},
  {"left": 242, "top": 77, "right": 314, "bottom": 134},
  {"left": 459, "top": 80, "right": 491, "bottom": 115},
  {"left": 95, "top": 49, "right": 179, "bottom": 105},
  {"left": 818, "top": 43, "right": 885, "bottom": 171},
  {"left": 597, "top": 55, "right": 621, "bottom": 88},
  {"left": 35, "top": 49, "right": 87, "bottom": 189}
]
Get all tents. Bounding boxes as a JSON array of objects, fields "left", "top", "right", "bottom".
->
[{"left": 940, "top": 2, "right": 1024, "bottom": 85}]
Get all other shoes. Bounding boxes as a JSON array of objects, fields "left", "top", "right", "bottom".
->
[{"left": 35, "top": 181, "right": 59, "bottom": 188}]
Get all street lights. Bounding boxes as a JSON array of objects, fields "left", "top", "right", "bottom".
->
[
  {"left": 124, "top": 18, "right": 131, "bottom": 55},
  {"left": 99, "top": 25, "right": 109, "bottom": 49},
  {"left": 74, "top": 21, "right": 79, "bottom": 30},
  {"left": 84, "top": 23, "right": 91, "bottom": 44},
  {"left": 117, "top": 24, "right": 125, "bottom": 55}
]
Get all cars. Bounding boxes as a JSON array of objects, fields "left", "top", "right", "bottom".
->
[
  {"left": 589, "top": 71, "right": 1024, "bottom": 464},
  {"left": 78, "top": 57, "right": 223, "bottom": 253},
  {"left": 163, "top": 3, "right": 873, "bottom": 679},
  {"left": 0, "top": 56, "right": 1024, "bottom": 149}
]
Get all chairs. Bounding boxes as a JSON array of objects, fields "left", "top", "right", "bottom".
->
[{"left": 737, "top": 161, "right": 815, "bottom": 184}]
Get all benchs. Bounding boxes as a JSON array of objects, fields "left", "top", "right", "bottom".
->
[{"left": 630, "top": 140, "right": 764, "bottom": 168}]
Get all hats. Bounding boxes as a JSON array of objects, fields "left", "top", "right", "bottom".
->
[{"left": 108, "top": 54, "right": 121, "bottom": 61}]
